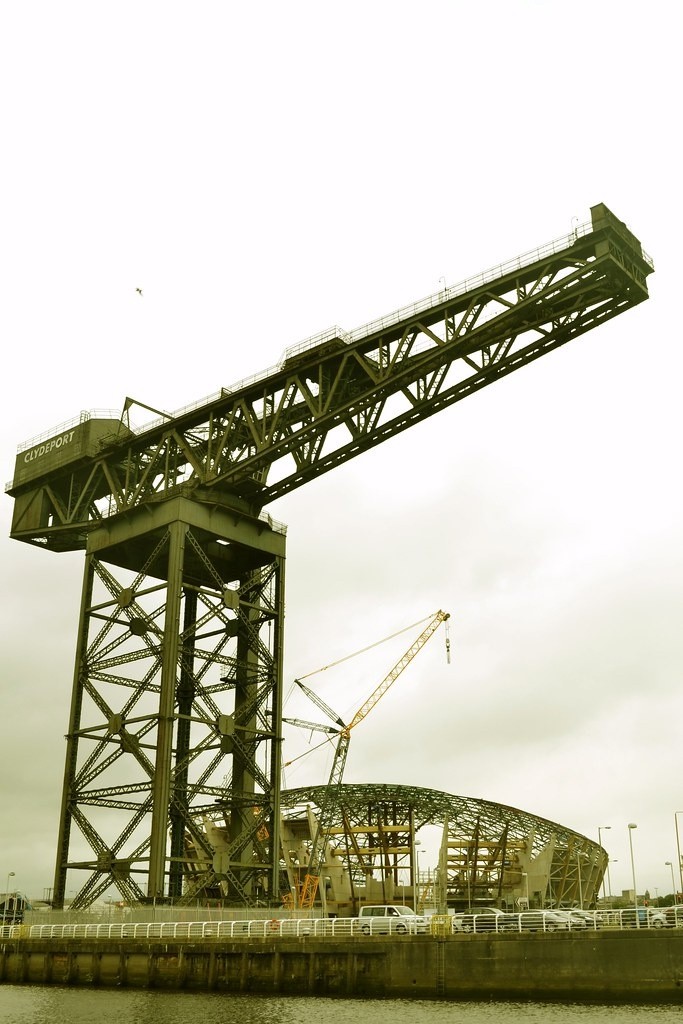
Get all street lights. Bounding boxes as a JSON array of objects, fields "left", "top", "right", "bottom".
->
[
  {"left": 627, "top": 821, "right": 641, "bottom": 930},
  {"left": 674, "top": 809, "right": 683, "bottom": 891},
  {"left": 665, "top": 860, "right": 677, "bottom": 904},
  {"left": 607, "top": 858, "right": 619, "bottom": 906},
  {"left": 598, "top": 823, "right": 611, "bottom": 907}
]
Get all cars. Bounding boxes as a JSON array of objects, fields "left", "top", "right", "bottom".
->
[
  {"left": 523, "top": 907, "right": 604, "bottom": 932},
  {"left": 617, "top": 902, "right": 663, "bottom": 927},
  {"left": 649, "top": 905, "right": 682, "bottom": 929}
]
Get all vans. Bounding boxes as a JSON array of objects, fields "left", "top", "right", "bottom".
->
[
  {"left": 459, "top": 907, "right": 516, "bottom": 933},
  {"left": 358, "top": 905, "right": 430, "bottom": 934}
]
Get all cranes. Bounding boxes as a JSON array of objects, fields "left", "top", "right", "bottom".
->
[{"left": 282, "top": 605, "right": 451, "bottom": 916}]
